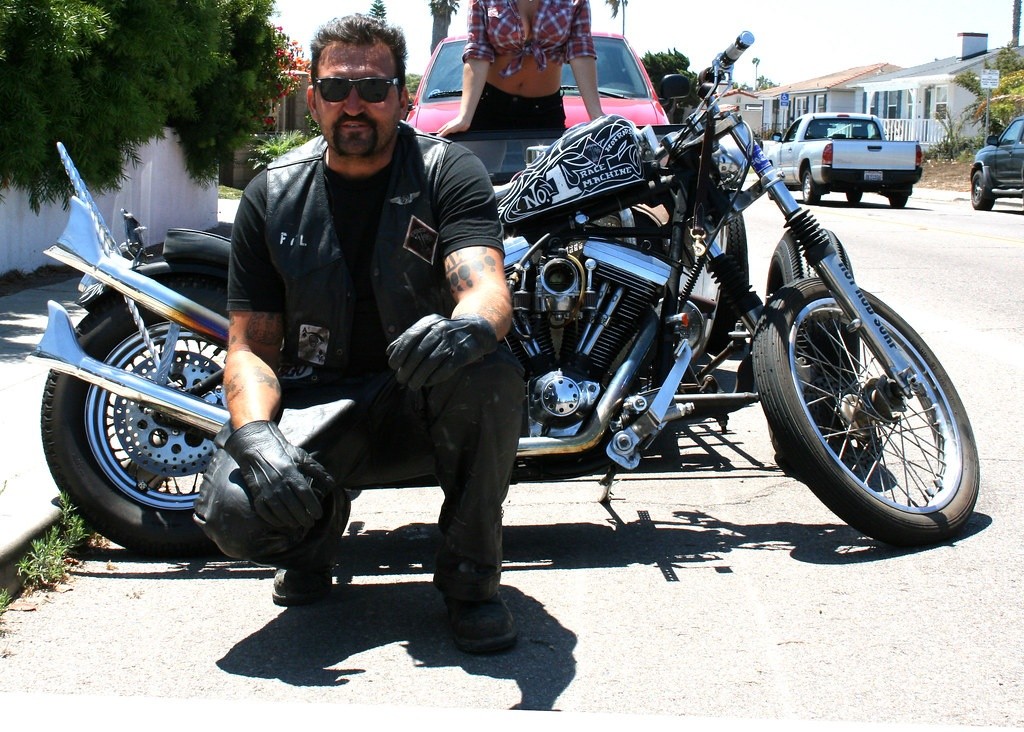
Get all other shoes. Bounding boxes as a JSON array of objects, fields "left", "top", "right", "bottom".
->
[
  {"left": 442, "top": 591, "right": 518, "bottom": 653},
  {"left": 271, "top": 542, "right": 327, "bottom": 606}
]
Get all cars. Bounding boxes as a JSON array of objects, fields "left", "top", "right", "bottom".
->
[{"left": 400, "top": 32, "right": 670, "bottom": 176}]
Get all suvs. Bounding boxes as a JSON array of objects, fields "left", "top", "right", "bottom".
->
[{"left": 970, "top": 116, "right": 1024, "bottom": 209}]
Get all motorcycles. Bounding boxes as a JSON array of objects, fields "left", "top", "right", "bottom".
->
[{"left": 34, "top": 28, "right": 983, "bottom": 559}]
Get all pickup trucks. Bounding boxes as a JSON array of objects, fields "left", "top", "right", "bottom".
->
[{"left": 761, "top": 112, "right": 926, "bottom": 208}]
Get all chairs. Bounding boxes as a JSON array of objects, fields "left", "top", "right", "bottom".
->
[
  {"left": 852, "top": 127, "right": 869, "bottom": 140},
  {"left": 812, "top": 126, "right": 828, "bottom": 138}
]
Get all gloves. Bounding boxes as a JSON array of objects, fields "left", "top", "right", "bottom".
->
[
  {"left": 387, "top": 314, "right": 499, "bottom": 391},
  {"left": 224, "top": 420, "right": 334, "bottom": 528}
]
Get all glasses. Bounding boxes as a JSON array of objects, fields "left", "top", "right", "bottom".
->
[{"left": 313, "top": 75, "right": 399, "bottom": 103}]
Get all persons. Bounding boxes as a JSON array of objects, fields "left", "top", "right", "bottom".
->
[
  {"left": 223, "top": 15, "right": 528, "bottom": 652},
  {"left": 434, "top": 0, "right": 607, "bottom": 137}
]
